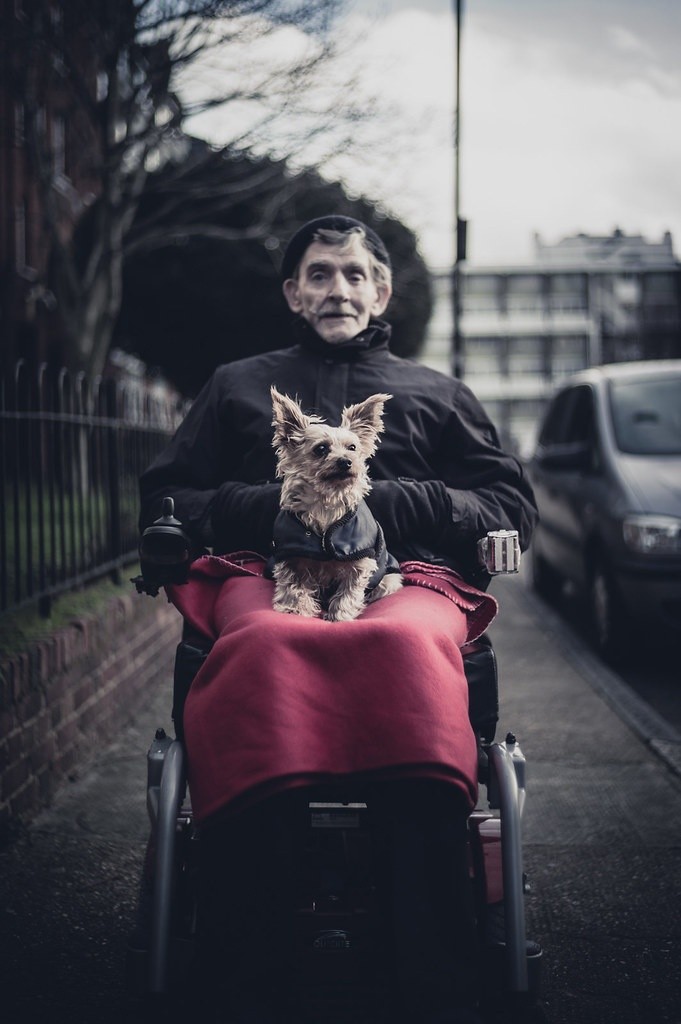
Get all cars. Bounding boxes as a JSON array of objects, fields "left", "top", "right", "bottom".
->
[{"left": 524, "top": 353, "right": 681, "bottom": 664}]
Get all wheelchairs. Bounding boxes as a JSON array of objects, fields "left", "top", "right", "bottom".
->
[{"left": 120, "top": 493, "right": 547, "bottom": 1023}]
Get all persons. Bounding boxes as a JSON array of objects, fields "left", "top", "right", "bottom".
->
[{"left": 137, "top": 215, "right": 544, "bottom": 960}]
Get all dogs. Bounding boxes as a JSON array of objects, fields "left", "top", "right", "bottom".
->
[{"left": 269, "top": 385, "right": 403, "bottom": 624}]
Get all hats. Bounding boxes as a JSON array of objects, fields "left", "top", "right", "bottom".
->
[{"left": 280, "top": 215, "right": 391, "bottom": 283}]
